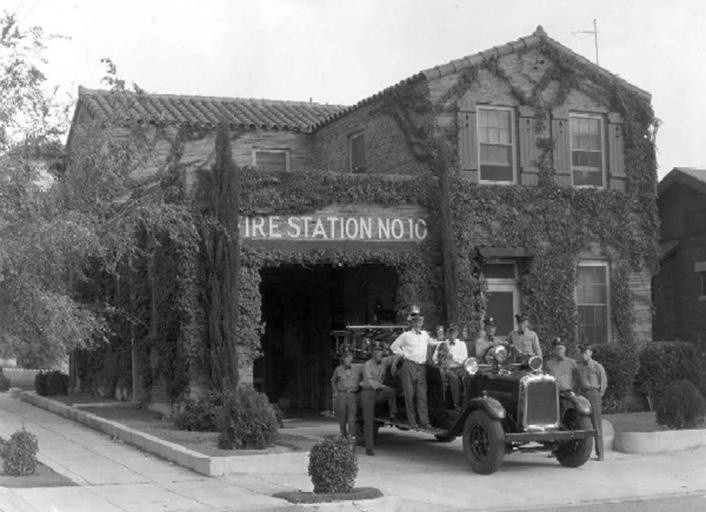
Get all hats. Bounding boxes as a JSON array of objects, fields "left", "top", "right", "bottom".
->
[
  {"left": 577, "top": 344, "right": 591, "bottom": 353},
  {"left": 408, "top": 313, "right": 424, "bottom": 321},
  {"left": 483, "top": 318, "right": 498, "bottom": 327},
  {"left": 551, "top": 337, "right": 565, "bottom": 348},
  {"left": 372, "top": 342, "right": 384, "bottom": 350},
  {"left": 515, "top": 313, "right": 528, "bottom": 323}
]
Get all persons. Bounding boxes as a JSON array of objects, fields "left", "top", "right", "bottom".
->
[
  {"left": 330, "top": 352, "right": 363, "bottom": 440},
  {"left": 360, "top": 348, "right": 401, "bottom": 456},
  {"left": 390, "top": 313, "right": 442, "bottom": 430},
  {"left": 427, "top": 325, "right": 446, "bottom": 363},
  {"left": 475, "top": 317, "right": 509, "bottom": 363},
  {"left": 505, "top": 312, "right": 542, "bottom": 362},
  {"left": 433, "top": 327, "right": 468, "bottom": 412},
  {"left": 544, "top": 337, "right": 578, "bottom": 458},
  {"left": 576, "top": 344, "right": 608, "bottom": 461}
]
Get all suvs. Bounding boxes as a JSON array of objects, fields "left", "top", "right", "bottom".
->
[{"left": 328, "top": 323, "right": 596, "bottom": 475}]
